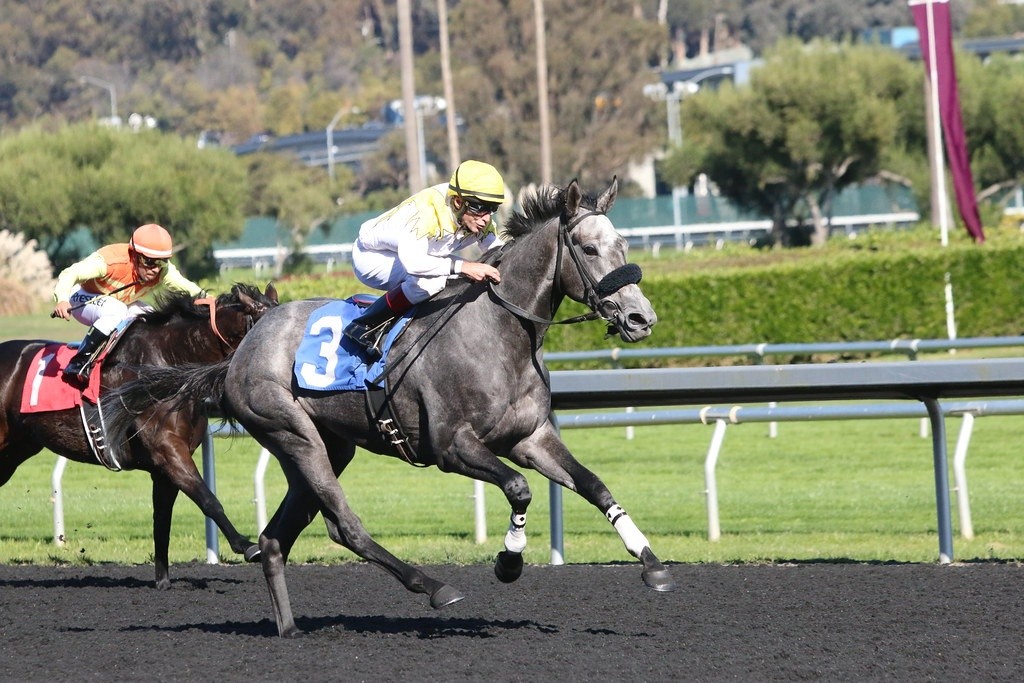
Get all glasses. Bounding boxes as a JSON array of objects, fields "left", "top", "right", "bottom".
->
[
  {"left": 465, "top": 200, "right": 498, "bottom": 214},
  {"left": 137, "top": 254, "right": 168, "bottom": 267}
]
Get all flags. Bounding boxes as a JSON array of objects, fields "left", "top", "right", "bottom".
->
[{"left": 909, "top": 0, "right": 986, "bottom": 242}]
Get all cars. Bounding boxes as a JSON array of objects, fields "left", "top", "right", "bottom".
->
[{"left": 744, "top": 222, "right": 819, "bottom": 251}]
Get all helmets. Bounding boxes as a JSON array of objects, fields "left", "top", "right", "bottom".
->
[
  {"left": 447, "top": 160, "right": 508, "bottom": 203},
  {"left": 129, "top": 224, "right": 172, "bottom": 258}
]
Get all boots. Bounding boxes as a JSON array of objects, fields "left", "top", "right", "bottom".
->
[
  {"left": 342, "top": 284, "right": 415, "bottom": 361},
  {"left": 64, "top": 326, "right": 110, "bottom": 389}
]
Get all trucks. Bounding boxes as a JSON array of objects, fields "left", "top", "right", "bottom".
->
[{"left": 641, "top": 59, "right": 759, "bottom": 105}]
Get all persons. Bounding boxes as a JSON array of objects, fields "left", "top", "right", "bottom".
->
[
  {"left": 342, "top": 160, "right": 504, "bottom": 356},
  {"left": 54, "top": 224, "right": 212, "bottom": 383}
]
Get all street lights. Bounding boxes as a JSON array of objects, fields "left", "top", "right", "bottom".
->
[
  {"left": 386, "top": 95, "right": 448, "bottom": 191},
  {"left": 639, "top": 76, "right": 700, "bottom": 250},
  {"left": 323, "top": 105, "right": 360, "bottom": 183},
  {"left": 74, "top": 73, "right": 117, "bottom": 121}
]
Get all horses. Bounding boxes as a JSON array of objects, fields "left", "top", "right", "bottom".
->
[{"left": 1, "top": 171, "right": 678, "bottom": 637}]
